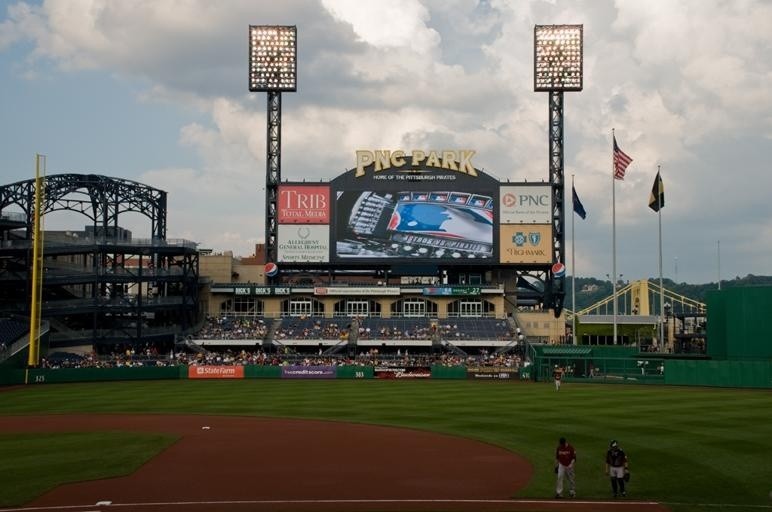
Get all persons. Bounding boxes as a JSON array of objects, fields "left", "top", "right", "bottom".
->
[
  {"left": 0, "top": 342, "right": 8, "bottom": 361},
  {"left": 605, "top": 440, "right": 628, "bottom": 498},
  {"left": 556, "top": 438, "right": 576, "bottom": 498}
]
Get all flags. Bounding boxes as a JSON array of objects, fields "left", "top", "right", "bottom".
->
[
  {"left": 573, "top": 187, "right": 586, "bottom": 219},
  {"left": 649, "top": 171, "right": 664, "bottom": 211},
  {"left": 613, "top": 135, "right": 632, "bottom": 180}
]
